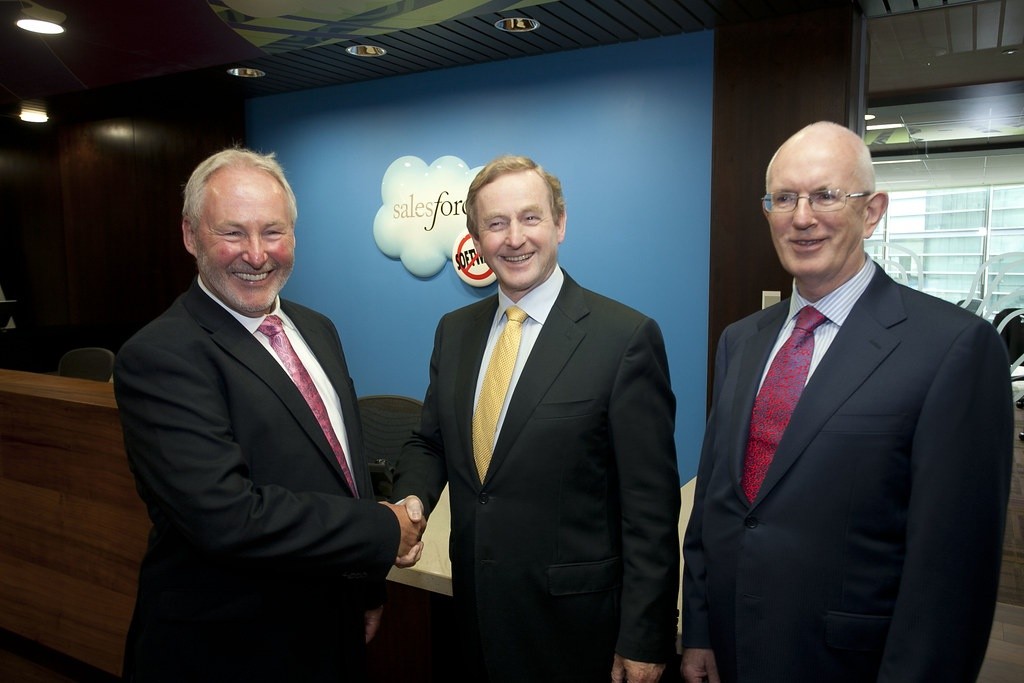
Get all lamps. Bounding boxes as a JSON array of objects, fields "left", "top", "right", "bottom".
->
[{"left": 19, "top": 98, "right": 50, "bottom": 123}]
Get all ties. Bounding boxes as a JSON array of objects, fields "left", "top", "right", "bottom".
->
[
  {"left": 256, "top": 314, "right": 358, "bottom": 499},
  {"left": 739, "top": 305, "right": 826, "bottom": 502},
  {"left": 472, "top": 304, "right": 530, "bottom": 485}
]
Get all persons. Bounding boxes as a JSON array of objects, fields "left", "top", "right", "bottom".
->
[
  {"left": 681, "top": 121, "right": 1015, "bottom": 683},
  {"left": 393, "top": 155, "right": 680, "bottom": 683},
  {"left": 113, "top": 144, "right": 425, "bottom": 683}
]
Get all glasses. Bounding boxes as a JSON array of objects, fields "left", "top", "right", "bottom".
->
[{"left": 758, "top": 188, "right": 873, "bottom": 214}]
